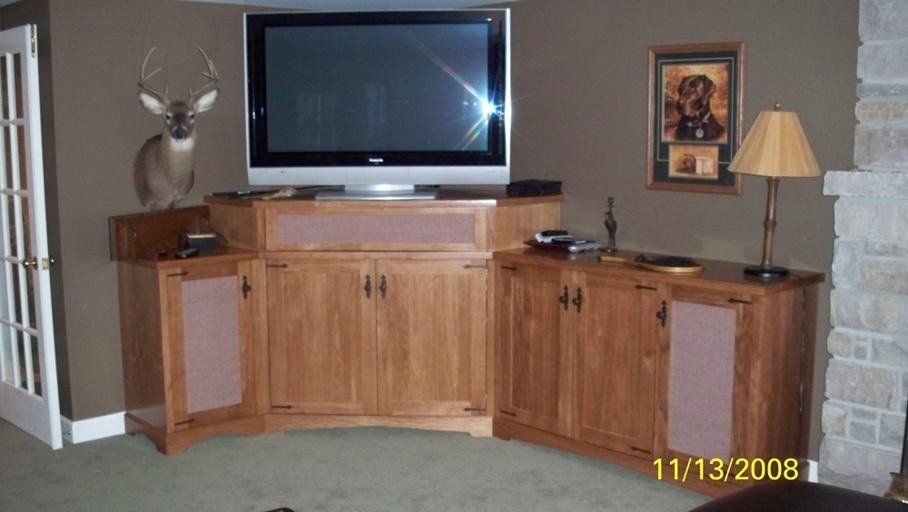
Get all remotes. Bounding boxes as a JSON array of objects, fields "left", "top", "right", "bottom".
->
[{"left": 174, "top": 246, "right": 199, "bottom": 258}]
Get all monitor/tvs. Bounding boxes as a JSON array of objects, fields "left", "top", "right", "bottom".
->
[{"left": 242, "top": 7, "right": 513, "bottom": 200}]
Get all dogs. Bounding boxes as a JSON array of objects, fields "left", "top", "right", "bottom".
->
[{"left": 671, "top": 73, "right": 725, "bottom": 142}]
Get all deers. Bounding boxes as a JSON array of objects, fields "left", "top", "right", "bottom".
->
[{"left": 133, "top": 43, "right": 220, "bottom": 210}]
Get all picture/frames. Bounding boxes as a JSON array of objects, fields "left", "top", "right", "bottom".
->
[{"left": 645, "top": 41, "right": 747, "bottom": 194}]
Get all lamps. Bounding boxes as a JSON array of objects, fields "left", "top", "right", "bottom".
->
[{"left": 725, "top": 101, "right": 825, "bottom": 284}]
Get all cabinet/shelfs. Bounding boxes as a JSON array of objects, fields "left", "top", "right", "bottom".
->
[
  {"left": 494, "top": 244, "right": 690, "bottom": 475},
  {"left": 206, "top": 187, "right": 562, "bottom": 438},
  {"left": 653, "top": 266, "right": 824, "bottom": 503},
  {"left": 114, "top": 204, "right": 256, "bottom": 456}
]
